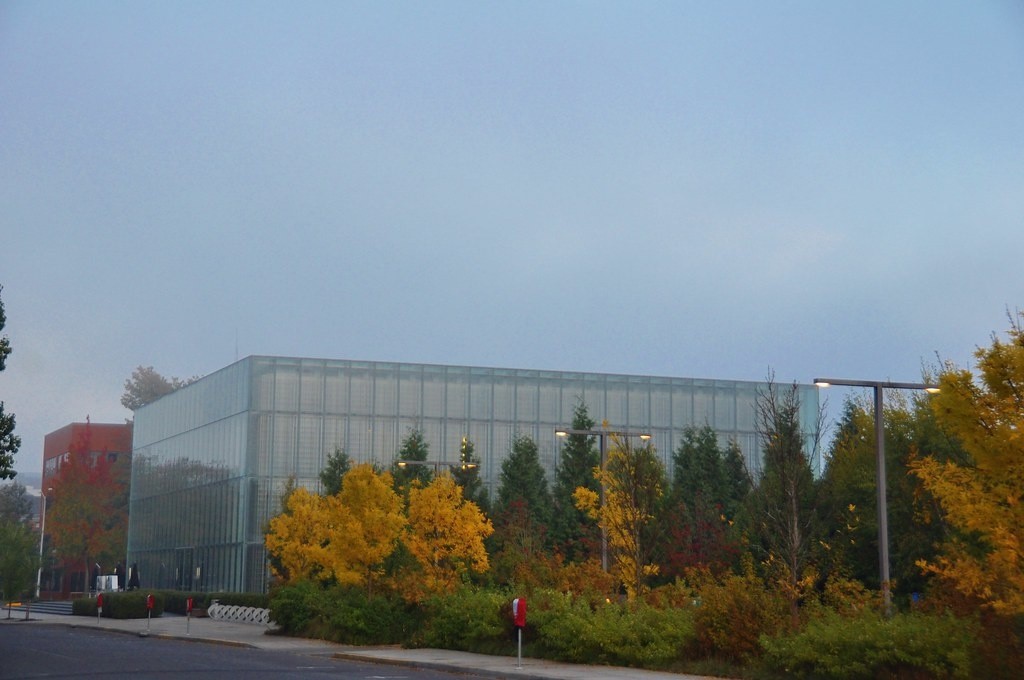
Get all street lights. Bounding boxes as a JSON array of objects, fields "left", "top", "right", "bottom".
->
[
  {"left": 37, "top": 486, "right": 54, "bottom": 598},
  {"left": 394, "top": 457, "right": 476, "bottom": 485},
  {"left": 555, "top": 428, "right": 651, "bottom": 572},
  {"left": 813, "top": 377, "right": 941, "bottom": 620}
]
